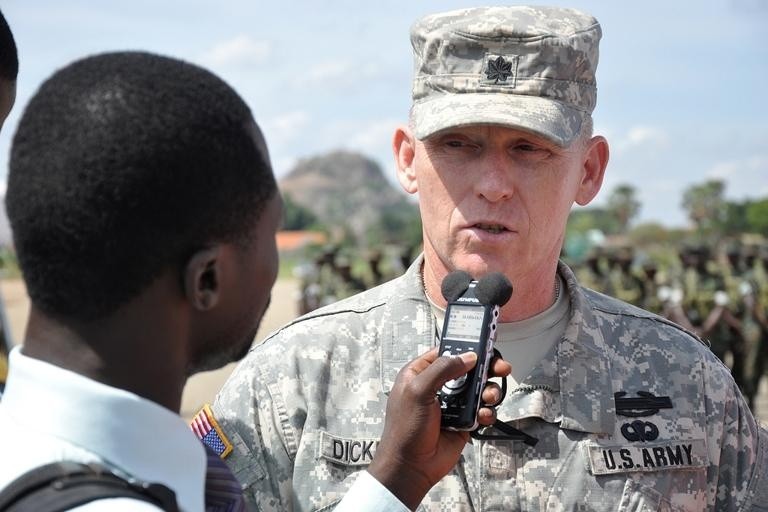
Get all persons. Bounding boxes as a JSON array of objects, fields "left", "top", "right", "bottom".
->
[
  {"left": 188, "top": 3, "right": 768, "bottom": 511},
  {"left": 0, "top": 10, "right": 19, "bottom": 402},
  {"left": 1, "top": 51, "right": 514, "bottom": 512},
  {"left": 297, "top": 240, "right": 413, "bottom": 316},
  {"left": 583, "top": 238, "right": 766, "bottom": 412}
]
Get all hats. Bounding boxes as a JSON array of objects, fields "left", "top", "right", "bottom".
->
[{"left": 409, "top": 4, "right": 602, "bottom": 149}]
